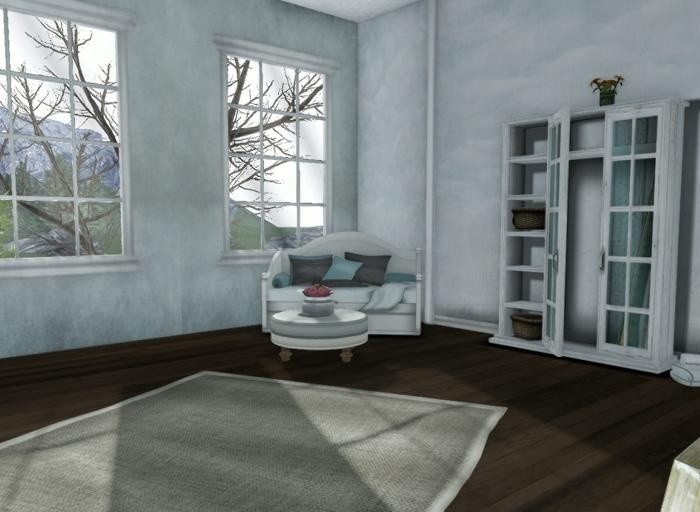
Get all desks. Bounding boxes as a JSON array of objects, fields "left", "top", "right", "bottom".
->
[{"left": 659, "top": 438, "right": 700, "bottom": 512}]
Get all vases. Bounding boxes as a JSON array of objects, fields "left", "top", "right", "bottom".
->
[
  {"left": 599, "top": 91, "right": 615, "bottom": 107},
  {"left": 302, "top": 296, "right": 334, "bottom": 316}
]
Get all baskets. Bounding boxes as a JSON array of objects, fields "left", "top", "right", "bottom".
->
[
  {"left": 512, "top": 208, "right": 545, "bottom": 229},
  {"left": 510, "top": 314, "right": 542, "bottom": 339}
]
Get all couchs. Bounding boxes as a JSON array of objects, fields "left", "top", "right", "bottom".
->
[{"left": 260, "top": 231, "right": 422, "bottom": 337}]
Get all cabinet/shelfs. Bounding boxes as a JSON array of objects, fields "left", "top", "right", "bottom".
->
[{"left": 486, "top": 96, "right": 691, "bottom": 375}]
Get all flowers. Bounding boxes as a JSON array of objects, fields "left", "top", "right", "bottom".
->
[
  {"left": 589, "top": 72, "right": 626, "bottom": 93},
  {"left": 301, "top": 283, "right": 334, "bottom": 298}
]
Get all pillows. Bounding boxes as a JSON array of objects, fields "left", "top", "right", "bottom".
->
[
  {"left": 343, "top": 251, "right": 393, "bottom": 287},
  {"left": 384, "top": 272, "right": 415, "bottom": 283},
  {"left": 273, "top": 272, "right": 290, "bottom": 287},
  {"left": 288, "top": 253, "right": 333, "bottom": 285},
  {"left": 322, "top": 256, "right": 363, "bottom": 280},
  {"left": 290, "top": 257, "right": 332, "bottom": 285}
]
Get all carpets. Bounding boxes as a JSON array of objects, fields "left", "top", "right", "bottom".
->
[{"left": 0, "top": 371, "right": 509, "bottom": 511}]
